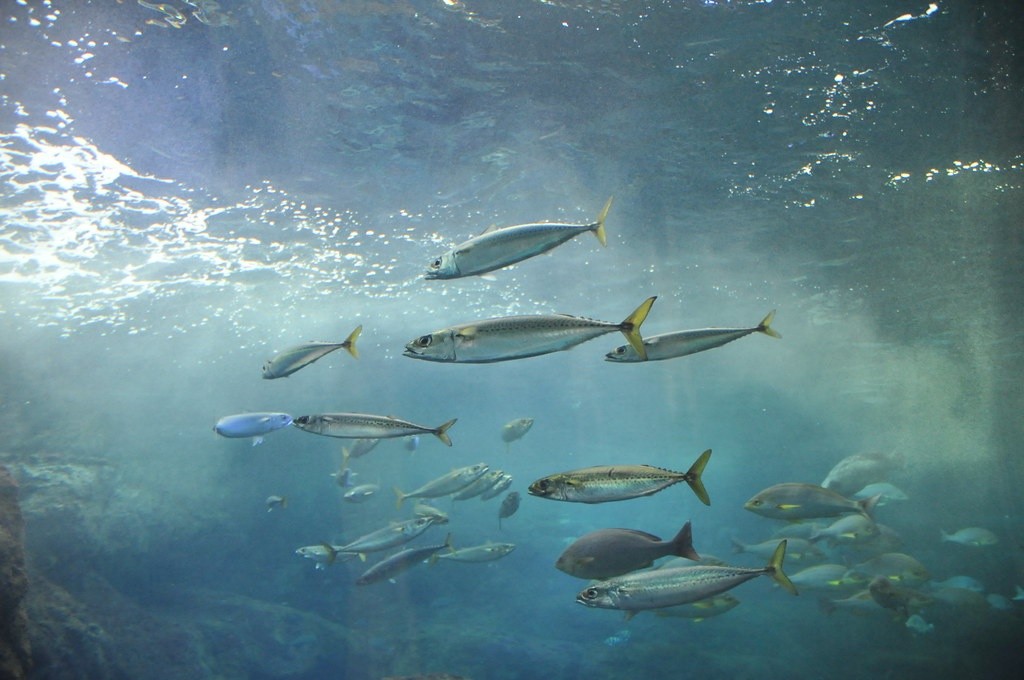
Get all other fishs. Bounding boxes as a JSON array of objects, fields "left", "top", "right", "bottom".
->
[{"left": 212, "top": 196, "right": 1024, "bottom": 641}]
